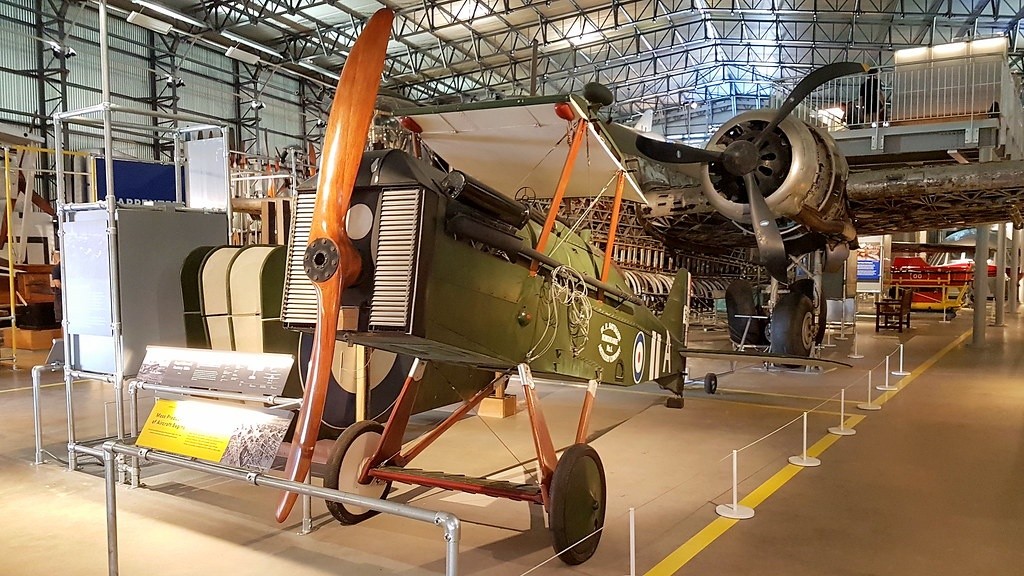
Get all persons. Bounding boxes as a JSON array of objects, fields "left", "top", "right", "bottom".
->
[
  {"left": 860, "top": 68, "right": 886, "bottom": 124},
  {"left": 52, "top": 248, "right": 63, "bottom": 341},
  {"left": 839, "top": 101, "right": 856, "bottom": 126}
]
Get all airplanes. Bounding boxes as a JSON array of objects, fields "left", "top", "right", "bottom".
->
[{"left": 274, "top": 6, "right": 1024, "bottom": 576}]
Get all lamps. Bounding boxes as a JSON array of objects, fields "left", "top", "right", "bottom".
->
[
  {"left": 251, "top": 100, "right": 258, "bottom": 110},
  {"left": 165, "top": 73, "right": 173, "bottom": 87},
  {"left": 174, "top": 76, "right": 187, "bottom": 88},
  {"left": 64, "top": 46, "right": 78, "bottom": 59},
  {"left": 317, "top": 118, "right": 322, "bottom": 127},
  {"left": 258, "top": 101, "right": 266, "bottom": 110},
  {"left": 50, "top": 42, "right": 61, "bottom": 59},
  {"left": 322, "top": 120, "right": 328, "bottom": 128}
]
